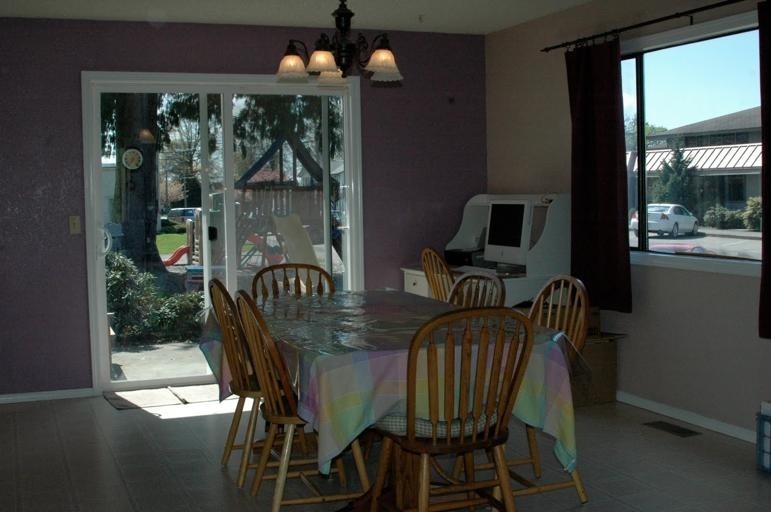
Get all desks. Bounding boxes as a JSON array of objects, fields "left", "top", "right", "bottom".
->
[
  {"left": 395, "top": 260, "right": 572, "bottom": 314},
  {"left": 235, "top": 288, "right": 587, "bottom": 511}
]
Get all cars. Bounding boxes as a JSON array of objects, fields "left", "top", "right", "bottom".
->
[
  {"left": 631, "top": 203, "right": 698, "bottom": 239},
  {"left": 162, "top": 208, "right": 201, "bottom": 233}
]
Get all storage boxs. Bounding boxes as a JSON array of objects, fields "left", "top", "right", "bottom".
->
[{"left": 517, "top": 304, "right": 628, "bottom": 409}]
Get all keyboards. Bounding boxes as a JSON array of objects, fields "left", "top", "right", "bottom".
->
[{"left": 453, "top": 265, "right": 496, "bottom": 277}]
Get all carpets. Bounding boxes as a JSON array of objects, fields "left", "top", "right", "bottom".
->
[{"left": 102, "top": 383, "right": 239, "bottom": 410}]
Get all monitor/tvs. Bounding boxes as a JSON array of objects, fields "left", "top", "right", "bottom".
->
[{"left": 484, "top": 199, "right": 530, "bottom": 272}]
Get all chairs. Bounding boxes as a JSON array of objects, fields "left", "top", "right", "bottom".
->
[
  {"left": 250, "top": 262, "right": 338, "bottom": 299},
  {"left": 490, "top": 274, "right": 588, "bottom": 507},
  {"left": 443, "top": 270, "right": 508, "bottom": 312},
  {"left": 418, "top": 246, "right": 458, "bottom": 303},
  {"left": 210, "top": 277, "right": 347, "bottom": 487},
  {"left": 230, "top": 286, "right": 372, "bottom": 511}
]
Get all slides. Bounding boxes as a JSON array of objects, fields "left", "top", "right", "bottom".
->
[
  {"left": 240, "top": 228, "right": 283, "bottom": 269},
  {"left": 161, "top": 244, "right": 189, "bottom": 267}
]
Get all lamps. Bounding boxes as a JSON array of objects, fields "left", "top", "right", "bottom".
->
[{"left": 275, "top": 0, "right": 404, "bottom": 82}]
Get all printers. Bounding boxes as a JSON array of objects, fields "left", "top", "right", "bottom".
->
[{"left": 444, "top": 227, "right": 497, "bottom": 268}]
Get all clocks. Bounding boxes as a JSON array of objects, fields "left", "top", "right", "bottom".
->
[{"left": 121, "top": 147, "right": 144, "bottom": 171}]
